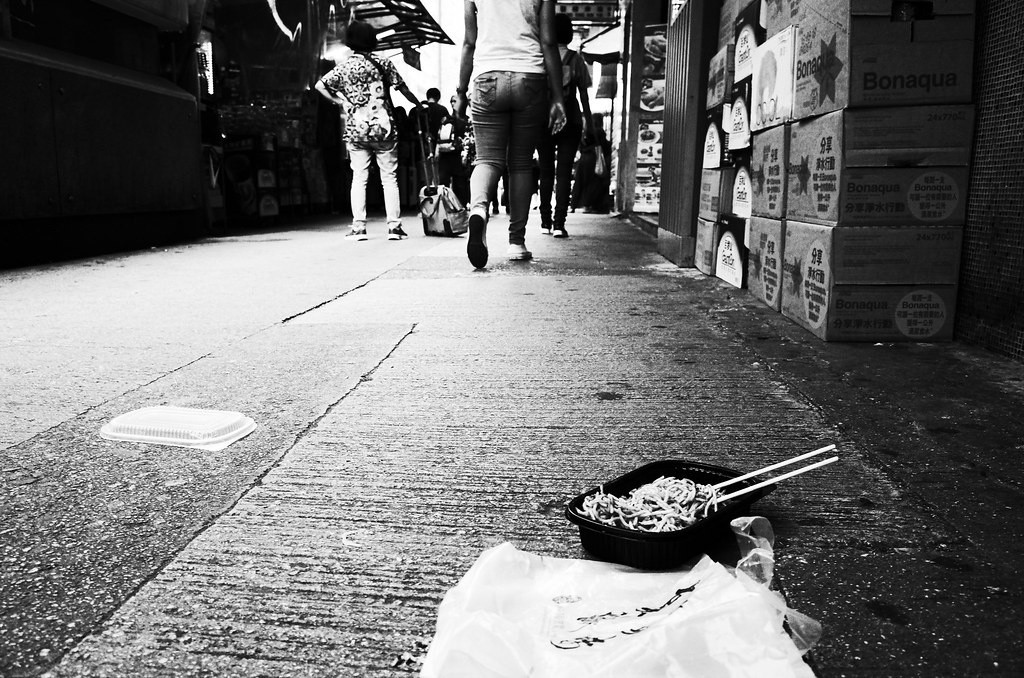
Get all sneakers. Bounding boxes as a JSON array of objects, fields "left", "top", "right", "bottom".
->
[
  {"left": 387, "top": 222, "right": 410, "bottom": 240},
  {"left": 345, "top": 224, "right": 367, "bottom": 240}
]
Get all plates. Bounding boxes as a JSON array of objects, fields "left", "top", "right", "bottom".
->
[
  {"left": 638, "top": 130, "right": 661, "bottom": 143},
  {"left": 640, "top": 80, "right": 665, "bottom": 111},
  {"left": 645, "top": 35, "right": 666, "bottom": 57}
]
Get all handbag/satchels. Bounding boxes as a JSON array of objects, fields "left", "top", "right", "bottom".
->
[{"left": 418, "top": 515, "right": 819, "bottom": 678}]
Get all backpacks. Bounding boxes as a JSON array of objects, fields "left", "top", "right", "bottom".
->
[{"left": 546, "top": 48, "right": 577, "bottom": 104}]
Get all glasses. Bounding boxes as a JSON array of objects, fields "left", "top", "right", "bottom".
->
[{"left": 450, "top": 100, "right": 456, "bottom": 102}]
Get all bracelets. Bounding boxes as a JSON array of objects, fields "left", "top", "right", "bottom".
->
[{"left": 456, "top": 87, "right": 468, "bottom": 92}]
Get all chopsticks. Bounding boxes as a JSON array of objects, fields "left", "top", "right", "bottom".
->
[{"left": 711, "top": 445, "right": 839, "bottom": 505}]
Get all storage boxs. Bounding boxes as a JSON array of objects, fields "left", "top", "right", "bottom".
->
[
  {"left": 0, "top": 55, "right": 203, "bottom": 221},
  {"left": 630, "top": 24, "right": 667, "bottom": 214},
  {"left": 692, "top": 0, "right": 978, "bottom": 342}
]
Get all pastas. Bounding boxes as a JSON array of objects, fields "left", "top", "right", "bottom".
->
[{"left": 576, "top": 473, "right": 728, "bottom": 533}]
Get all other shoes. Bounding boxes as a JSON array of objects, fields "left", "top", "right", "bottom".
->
[
  {"left": 540, "top": 218, "right": 553, "bottom": 234},
  {"left": 552, "top": 221, "right": 569, "bottom": 237},
  {"left": 466, "top": 209, "right": 489, "bottom": 268},
  {"left": 583, "top": 209, "right": 591, "bottom": 213},
  {"left": 508, "top": 243, "right": 532, "bottom": 260},
  {"left": 567, "top": 208, "right": 575, "bottom": 213}
]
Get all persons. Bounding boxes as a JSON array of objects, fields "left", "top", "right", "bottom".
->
[
  {"left": 392, "top": 88, "right": 470, "bottom": 211},
  {"left": 538, "top": 13, "right": 592, "bottom": 238},
  {"left": 492, "top": 176, "right": 510, "bottom": 214},
  {"left": 454, "top": 0, "right": 568, "bottom": 268},
  {"left": 567, "top": 113, "right": 610, "bottom": 214},
  {"left": 315, "top": 21, "right": 424, "bottom": 242}
]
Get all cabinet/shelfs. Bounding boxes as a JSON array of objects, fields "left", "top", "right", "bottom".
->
[{"left": 224, "top": 133, "right": 309, "bottom": 221}]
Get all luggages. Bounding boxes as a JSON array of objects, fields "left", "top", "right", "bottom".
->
[{"left": 415, "top": 106, "right": 469, "bottom": 236}]
